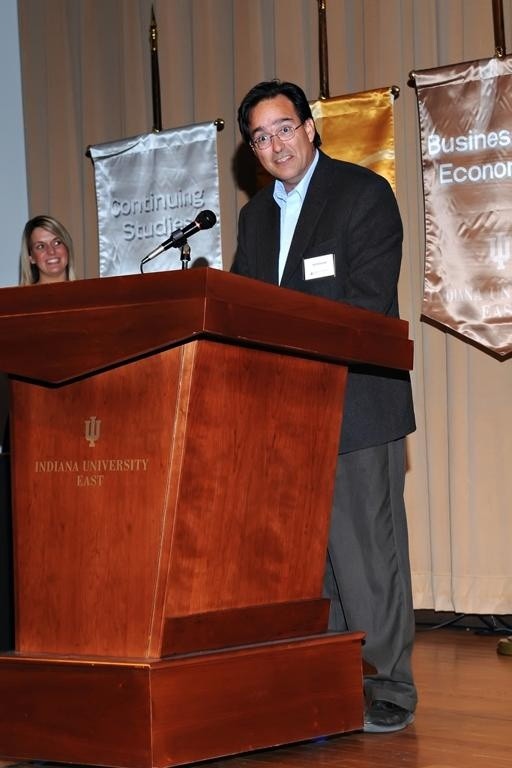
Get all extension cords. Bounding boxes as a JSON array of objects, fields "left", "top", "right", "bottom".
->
[{"left": 496, "top": 639, "right": 512, "bottom": 655}]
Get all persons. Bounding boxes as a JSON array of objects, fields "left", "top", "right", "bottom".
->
[
  {"left": 227, "top": 76, "right": 422, "bottom": 740},
  {"left": 16, "top": 214, "right": 79, "bottom": 287}
]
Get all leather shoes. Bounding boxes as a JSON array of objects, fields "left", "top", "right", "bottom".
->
[{"left": 363, "top": 701, "right": 415, "bottom": 732}]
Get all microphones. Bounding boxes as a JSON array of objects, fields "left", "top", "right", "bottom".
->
[{"left": 141, "top": 210, "right": 217, "bottom": 265}]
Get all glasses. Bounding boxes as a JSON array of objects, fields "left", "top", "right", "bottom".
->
[{"left": 251, "top": 123, "right": 305, "bottom": 150}]
